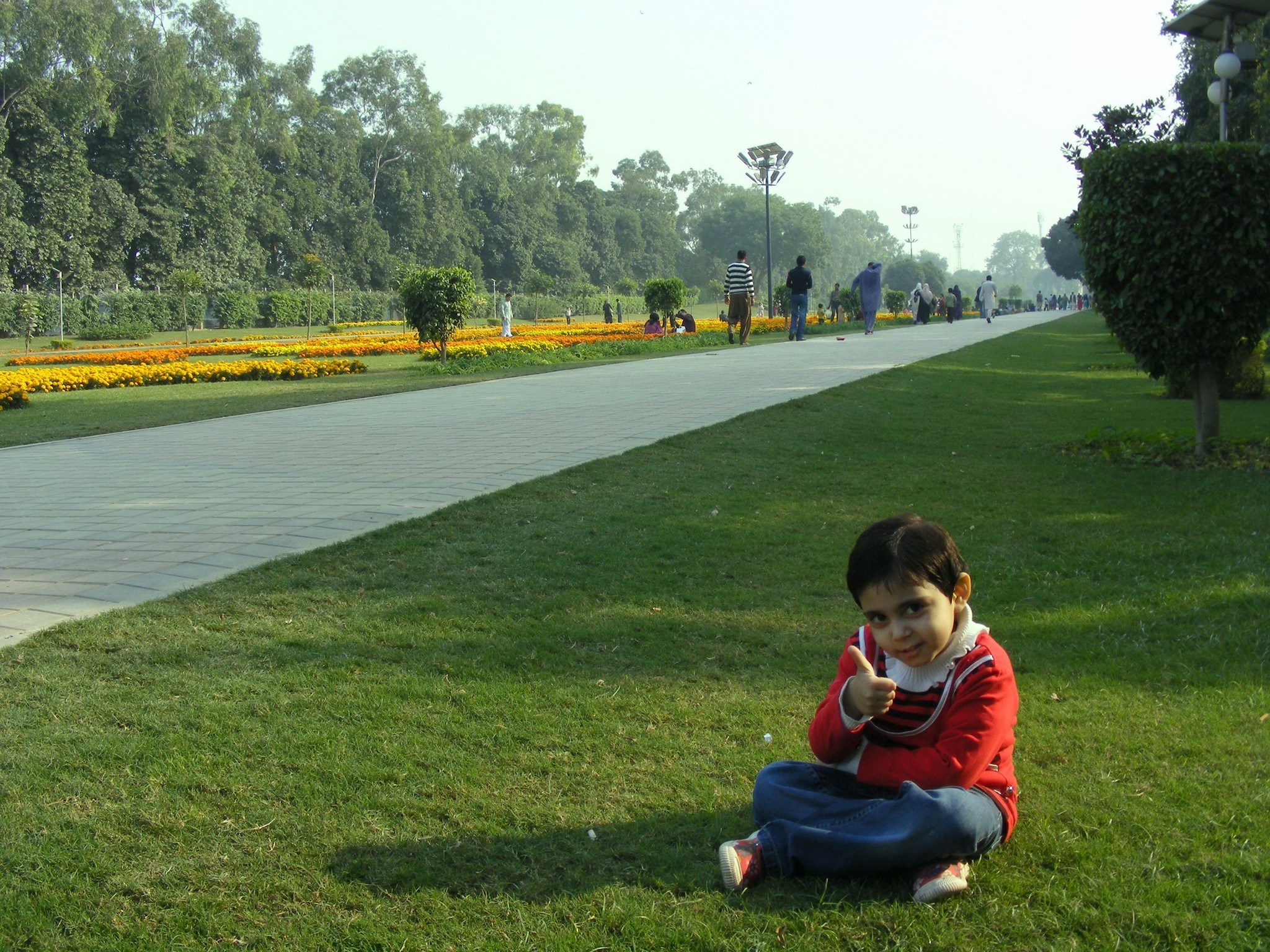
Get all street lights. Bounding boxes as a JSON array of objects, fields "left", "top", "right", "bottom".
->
[
  {"left": 901, "top": 205, "right": 919, "bottom": 259},
  {"left": 737, "top": 142, "right": 794, "bottom": 318},
  {"left": 1162, "top": 0, "right": 1270, "bottom": 141}
]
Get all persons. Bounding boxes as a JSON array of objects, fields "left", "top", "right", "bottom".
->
[
  {"left": 671, "top": 309, "right": 696, "bottom": 333},
  {"left": 849, "top": 262, "right": 883, "bottom": 335},
  {"left": 817, "top": 303, "right": 825, "bottom": 325},
  {"left": 565, "top": 307, "right": 571, "bottom": 324},
  {"left": 719, "top": 512, "right": 1020, "bottom": 903},
  {"left": 772, "top": 304, "right": 776, "bottom": 317},
  {"left": 786, "top": 255, "right": 812, "bottom": 341},
  {"left": 906, "top": 275, "right": 1013, "bottom": 326},
  {"left": 827, "top": 283, "right": 841, "bottom": 324},
  {"left": 500, "top": 293, "right": 514, "bottom": 337},
  {"left": 724, "top": 250, "right": 756, "bottom": 345},
  {"left": 1025, "top": 290, "right": 1095, "bottom": 313},
  {"left": 616, "top": 299, "right": 622, "bottom": 322},
  {"left": 719, "top": 310, "right": 728, "bottom": 321},
  {"left": 644, "top": 313, "right": 663, "bottom": 334},
  {"left": 757, "top": 302, "right": 764, "bottom": 317},
  {"left": 603, "top": 300, "right": 614, "bottom": 323}
]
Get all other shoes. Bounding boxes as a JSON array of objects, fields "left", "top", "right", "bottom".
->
[{"left": 724, "top": 315, "right": 995, "bottom": 347}]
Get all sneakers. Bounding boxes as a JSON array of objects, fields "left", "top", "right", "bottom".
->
[
  {"left": 912, "top": 860, "right": 969, "bottom": 902},
  {"left": 719, "top": 829, "right": 762, "bottom": 891}
]
